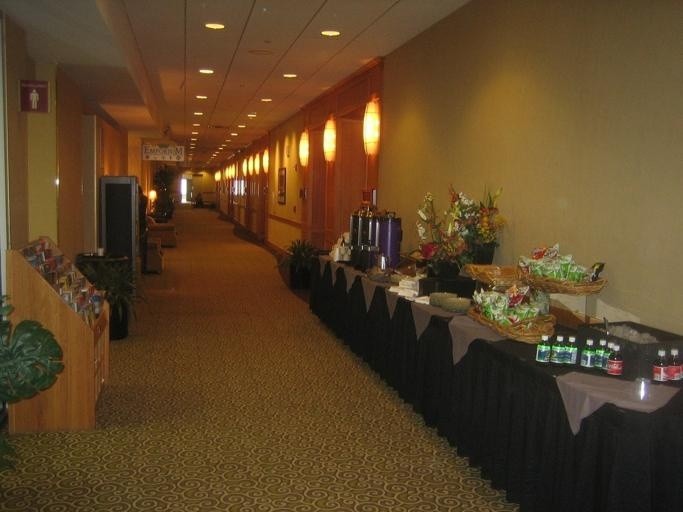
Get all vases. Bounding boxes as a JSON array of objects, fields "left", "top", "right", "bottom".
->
[
  {"left": 466, "top": 242, "right": 499, "bottom": 266},
  {"left": 427, "top": 260, "right": 460, "bottom": 279}
]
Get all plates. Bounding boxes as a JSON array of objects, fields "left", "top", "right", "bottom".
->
[
  {"left": 441, "top": 297, "right": 470, "bottom": 313},
  {"left": 429, "top": 293, "right": 457, "bottom": 306}
]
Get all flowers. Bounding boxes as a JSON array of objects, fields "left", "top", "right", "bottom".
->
[
  {"left": 443, "top": 182, "right": 509, "bottom": 244},
  {"left": 412, "top": 191, "right": 472, "bottom": 271}
]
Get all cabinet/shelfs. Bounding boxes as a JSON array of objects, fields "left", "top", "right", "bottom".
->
[{"left": 6, "top": 234, "right": 112, "bottom": 437}]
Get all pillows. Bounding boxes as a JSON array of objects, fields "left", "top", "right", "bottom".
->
[{"left": 146, "top": 215, "right": 156, "bottom": 224}]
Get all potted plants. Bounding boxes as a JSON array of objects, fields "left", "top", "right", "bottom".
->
[
  {"left": 78, "top": 259, "right": 146, "bottom": 340},
  {"left": 271, "top": 238, "right": 325, "bottom": 291}
]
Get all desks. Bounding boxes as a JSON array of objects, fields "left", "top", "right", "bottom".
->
[{"left": 308, "top": 251, "right": 683, "bottom": 512}]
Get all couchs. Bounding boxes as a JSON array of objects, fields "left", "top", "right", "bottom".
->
[
  {"left": 146, "top": 237, "right": 165, "bottom": 275},
  {"left": 145, "top": 217, "right": 177, "bottom": 247}
]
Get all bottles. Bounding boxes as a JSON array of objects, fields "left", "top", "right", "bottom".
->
[
  {"left": 653, "top": 350, "right": 667, "bottom": 383},
  {"left": 565, "top": 337, "right": 580, "bottom": 365},
  {"left": 608, "top": 345, "right": 623, "bottom": 376},
  {"left": 551, "top": 335, "right": 564, "bottom": 364},
  {"left": 581, "top": 339, "right": 595, "bottom": 367},
  {"left": 666, "top": 349, "right": 682, "bottom": 381},
  {"left": 378, "top": 253, "right": 386, "bottom": 270},
  {"left": 603, "top": 342, "right": 614, "bottom": 371},
  {"left": 535, "top": 335, "right": 550, "bottom": 362},
  {"left": 596, "top": 339, "right": 606, "bottom": 367}
]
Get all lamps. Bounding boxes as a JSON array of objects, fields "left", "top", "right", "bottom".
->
[
  {"left": 362, "top": 100, "right": 381, "bottom": 155},
  {"left": 298, "top": 131, "right": 309, "bottom": 168},
  {"left": 214, "top": 149, "right": 269, "bottom": 182},
  {"left": 322, "top": 119, "right": 337, "bottom": 163}
]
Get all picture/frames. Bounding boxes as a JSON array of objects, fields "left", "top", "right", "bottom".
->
[{"left": 277, "top": 168, "right": 286, "bottom": 204}]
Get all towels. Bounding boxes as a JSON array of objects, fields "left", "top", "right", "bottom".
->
[
  {"left": 555, "top": 370, "right": 681, "bottom": 437},
  {"left": 318, "top": 254, "right": 508, "bottom": 367}
]
